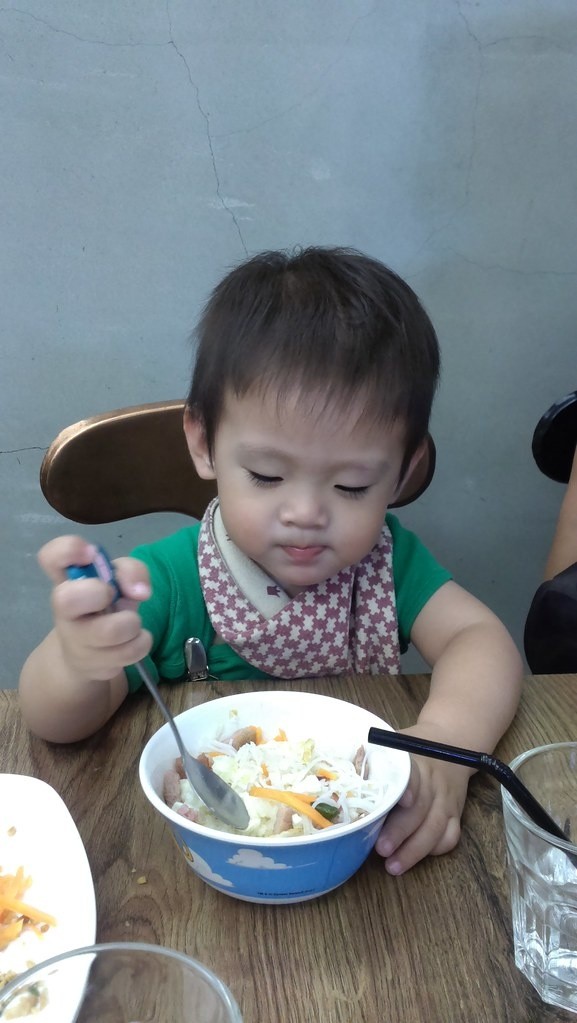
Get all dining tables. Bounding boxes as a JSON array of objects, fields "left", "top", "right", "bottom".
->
[{"left": 0, "top": 672, "right": 577, "bottom": 1023}]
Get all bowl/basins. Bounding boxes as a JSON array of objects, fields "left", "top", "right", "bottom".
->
[{"left": 138, "top": 690, "right": 411, "bottom": 907}]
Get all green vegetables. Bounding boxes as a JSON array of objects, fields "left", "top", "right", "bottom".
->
[{"left": 311, "top": 801, "right": 340, "bottom": 822}]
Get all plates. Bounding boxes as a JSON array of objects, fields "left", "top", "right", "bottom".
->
[{"left": 0, "top": 775, "right": 96, "bottom": 1021}]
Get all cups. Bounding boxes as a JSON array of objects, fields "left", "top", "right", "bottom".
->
[
  {"left": 501, "top": 742, "right": 575, "bottom": 1012},
  {"left": 0, "top": 946, "right": 244, "bottom": 1023}
]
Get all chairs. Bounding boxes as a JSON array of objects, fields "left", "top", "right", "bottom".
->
[{"left": 42, "top": 399, "right": 437, "bottom": 526}]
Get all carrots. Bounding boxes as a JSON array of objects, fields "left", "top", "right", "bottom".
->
[
  {"left": 247, "top": 727, "right": 336, "bottom": 828},
  {"left": 0, "top": 865, "right": 54, "bottom": 954}
]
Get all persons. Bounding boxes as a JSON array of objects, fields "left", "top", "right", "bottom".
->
[
  {"left": 524, "top": 451, "right": 577, "bottom": 675},
  {"left": 19, "top": 246, "right": 524, "bottom": 875}
]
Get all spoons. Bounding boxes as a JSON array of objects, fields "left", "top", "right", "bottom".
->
[{"left": 63, "top": 541, "right": 250, "bottom": 829}]
